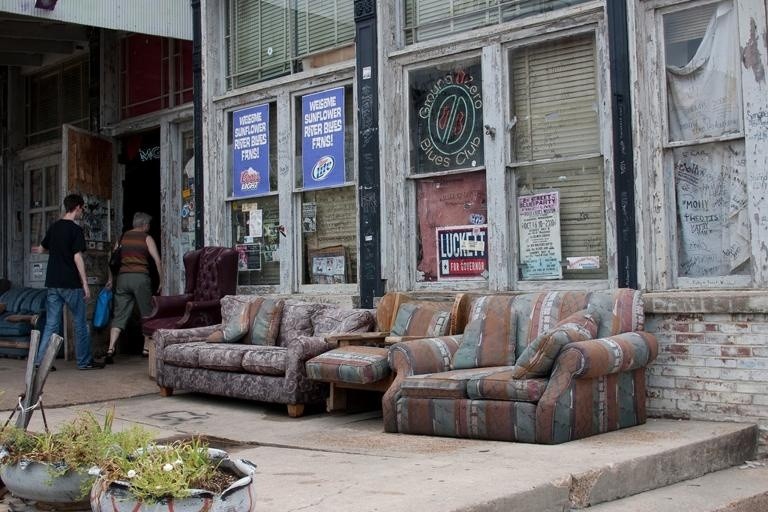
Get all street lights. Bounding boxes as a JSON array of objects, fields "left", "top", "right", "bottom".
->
[
  {"left": 107, "top": 245, "right": 122, "bottom": 275},
  {"left": 93, "top": 285, "right": 115, "bottom": 327}
]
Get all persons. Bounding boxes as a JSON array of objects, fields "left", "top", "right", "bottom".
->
[
  {"left": 105, "top": 211, "right": 165, "bottom": 365},
  {"left": 31, "top": 194, "right": 105, "bottom": 371}
]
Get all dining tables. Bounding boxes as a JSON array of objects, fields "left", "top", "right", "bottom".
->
[
  {"left": 90, "top": 427, "right": 260, "bottom": 512},
  {"left": 2, "top": 405, "right": 113, "bottom": 504}
]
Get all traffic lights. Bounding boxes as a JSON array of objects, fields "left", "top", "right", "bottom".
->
[
  {"left": 389, "top": 303, "right": 450, "bottom": 337},
  {"left": 513, "top": 310, "right": 598, "bottom": 377},
  {"left": 205, "top": 303, "right": 249, "bottom": 343},
  {"left": 251, "top": 299, "right": 285, "bottom": 346},
  {"left": 450, "top": 314, "right": 517, "bottom": 370}
]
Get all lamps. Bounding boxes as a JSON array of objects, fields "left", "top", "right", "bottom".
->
[
  {"left": 141, "top": 246, "right": 235, "bottom": 352},
  {"left": 304, "top": 291, "right": 469, "bottom": 414}
]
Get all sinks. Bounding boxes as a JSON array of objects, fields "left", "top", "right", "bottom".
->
[
  {"left": 0, "top": 287, "right": 47, "bottom": 359},
  {"left": 150, "top": 295, "right": 377, "bottom": 419},
  {"left": 382, "top": 287, "right": 658, "bottom": 446}
]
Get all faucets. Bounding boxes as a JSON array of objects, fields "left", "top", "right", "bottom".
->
[
  {"left": 106, "top": 347, "right": 116, "bottom": 360},
  {"left": 140, "top": 348, "right": 151, "bottom": 357}
]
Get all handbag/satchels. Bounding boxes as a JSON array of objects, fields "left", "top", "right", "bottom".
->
[{"left": 108, "top": 280, "right": 113, "bottom": 282}]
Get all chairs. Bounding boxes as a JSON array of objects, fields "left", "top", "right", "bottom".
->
[
  {"left": 34, "top": 363, "right": 56, "bottom": 372},
  {"left": 80, "top": 362, "right": 107, "bottom": 370}
]
